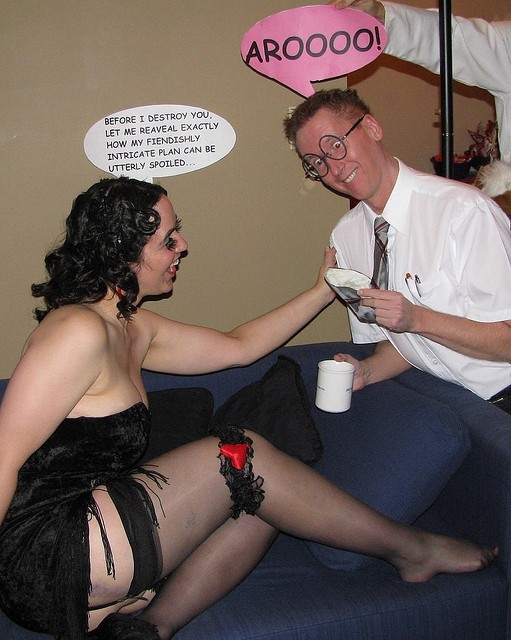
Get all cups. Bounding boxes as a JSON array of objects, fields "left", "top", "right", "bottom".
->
[{"left": 314, "top": 359, "right": 358, "bottom": 414}]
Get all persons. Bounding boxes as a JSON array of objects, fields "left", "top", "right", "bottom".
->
[
  {"left": 0, "top": 177, "right": 503, "bottom": 639},
  {"left": 282, "top": 88, "right": 511, "bottom": 574},
  {"left": 326, "top": 0, "right": 511, "bottom": 169}
]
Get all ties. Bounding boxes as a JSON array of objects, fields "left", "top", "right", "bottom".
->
[{"left": 324, "top": 218, "right": 391, "bottom": 323}]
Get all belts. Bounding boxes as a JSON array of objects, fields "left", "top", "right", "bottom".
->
[{"left": 490, "top": 391, "right": 511, "bottom": 416}]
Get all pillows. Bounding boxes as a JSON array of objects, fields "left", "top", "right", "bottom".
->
[{"left": 298, "top": 359, "right": 472, "bottom": 570}]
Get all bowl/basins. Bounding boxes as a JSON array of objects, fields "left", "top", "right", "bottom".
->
[{"left": 430, "top": 154, "right": 472, "bottom": 181}]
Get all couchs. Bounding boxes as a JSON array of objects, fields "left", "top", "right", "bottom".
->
[{"left": 0, "top": 340, "right": 510, "bottom": 639}]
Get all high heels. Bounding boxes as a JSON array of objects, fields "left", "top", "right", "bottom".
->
[{"left": 86, "top": 610, "right": 161, "bottom": 640}]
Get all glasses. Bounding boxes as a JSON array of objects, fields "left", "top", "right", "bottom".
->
[{"left": 303, "top": 114, "right": 365, "bottom": 182}]
